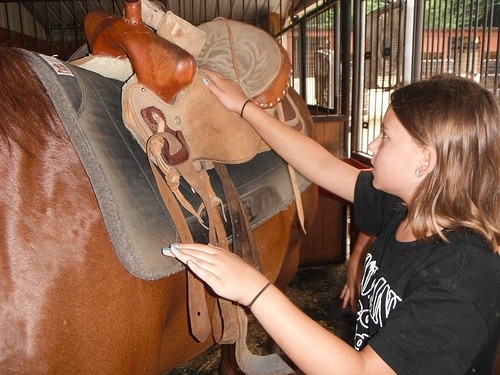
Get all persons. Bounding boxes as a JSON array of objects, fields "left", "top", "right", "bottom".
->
[{"left": 163, "top": 65, "right": 499, "bottom": 371}]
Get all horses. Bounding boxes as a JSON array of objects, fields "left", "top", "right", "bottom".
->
[{"left": 0, "top": 1, "right": 319, "bottom": 375}]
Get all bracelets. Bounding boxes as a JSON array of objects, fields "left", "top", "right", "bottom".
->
[
  {"left": 240, "top": 100, "right": 253, "bottom": 118},
  {"left": 247, "top": 282, "right": 272, "bottom": 309}
]
